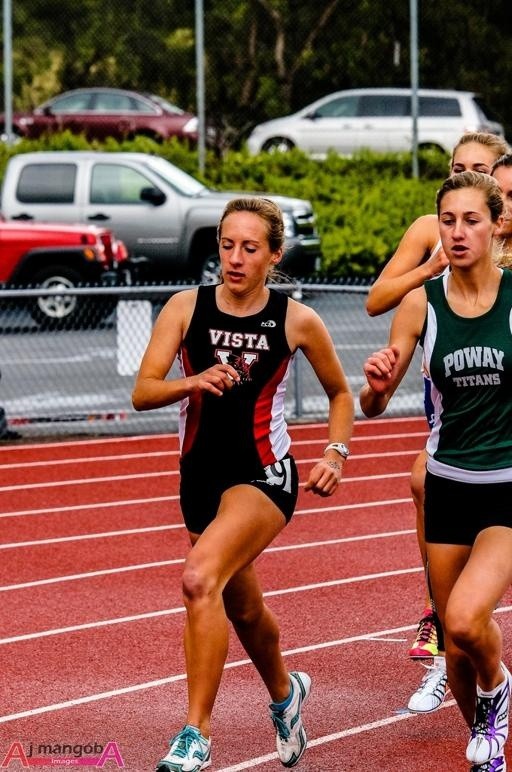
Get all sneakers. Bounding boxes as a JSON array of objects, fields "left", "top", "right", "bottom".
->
[
  {"left": 462, "top": 663, "right": 512, "bottom": 772},
  {"left": 154, "top": 725, "right": 213, "bottom": 772},
  {"left": 406, "top": 603, "right": 441, "bottom": 660},
  {"left": 407, "top": 655, "right": 451, "bottom": 715},
  {"left": 269, "top": 671, "right": 314, "bottom": 768}
]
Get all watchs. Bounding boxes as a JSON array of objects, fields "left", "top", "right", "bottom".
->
[{"left": 324, "top": 443, "right": 349, "bottom": 461}]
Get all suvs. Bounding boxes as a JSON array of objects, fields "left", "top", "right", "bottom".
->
[
  {"left": 0, "top": 146, "right": 322, "bottom": 284},
  {"left": 237, "top": 81, "right": 507, "bottom": 170},
  {"left": 0, "top": 204, "right": 137, "bottom": 335}
]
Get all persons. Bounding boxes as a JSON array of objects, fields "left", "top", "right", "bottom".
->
[
  {"left": 366, "top": 132, "right": 510, "bottom": 659},
  {"left": 132, "top": 198, "right": 354, "bottom": 770},
  {"left": 354, "top": 171, "right": 512, "bottom": 770},
  {"left": 376, "top": 150, "right": 512, "bottom": 714}
]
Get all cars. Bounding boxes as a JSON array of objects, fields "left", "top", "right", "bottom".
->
[{"left": 0, "top": 85, "right": 218, "bottom": 151}]
